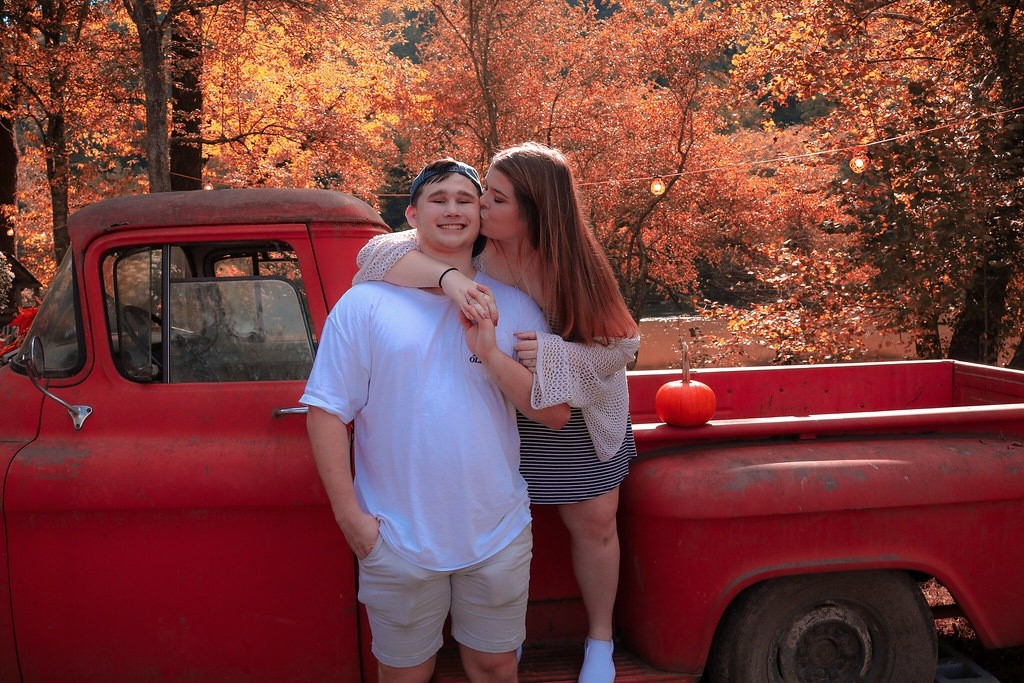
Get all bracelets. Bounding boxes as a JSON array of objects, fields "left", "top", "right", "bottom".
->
[{"left": 437, "top": 267, "right": 459, "bottom": 288}]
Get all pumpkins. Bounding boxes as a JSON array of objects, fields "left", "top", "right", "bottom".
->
[{"left": 655, "top": 340, "right": 716, "bottom": 427}]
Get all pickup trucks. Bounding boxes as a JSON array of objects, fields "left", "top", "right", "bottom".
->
[{"left": 0, "top": 188, "right": 1024, "bottom": 683}]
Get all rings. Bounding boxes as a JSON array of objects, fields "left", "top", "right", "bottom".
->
[
  {"left": 467, "top": 296, "right": 473, "bottom": 304},
  {"left": 530, "top": 360, "right": 534, "bottom": 367}
]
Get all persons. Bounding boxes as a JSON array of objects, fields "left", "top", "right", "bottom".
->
[
  {"left": 348, "top": 140, "right": 641, "bottom": 683},
  {"left": 298, "top": 159, "right": 569, "bottom": 683}
]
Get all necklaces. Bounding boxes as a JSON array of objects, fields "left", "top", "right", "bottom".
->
[{"left": 500, "top": 249, "right": 542, "bottom": 293}]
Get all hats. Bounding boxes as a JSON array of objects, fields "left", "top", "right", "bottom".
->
[{"left": 410, "top": 161, "right": 487, "bottom": 258}]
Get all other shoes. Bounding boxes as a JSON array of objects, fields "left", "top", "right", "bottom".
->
[{"left": 578, "top": 635, "right": 616, "bottom": 683}]
